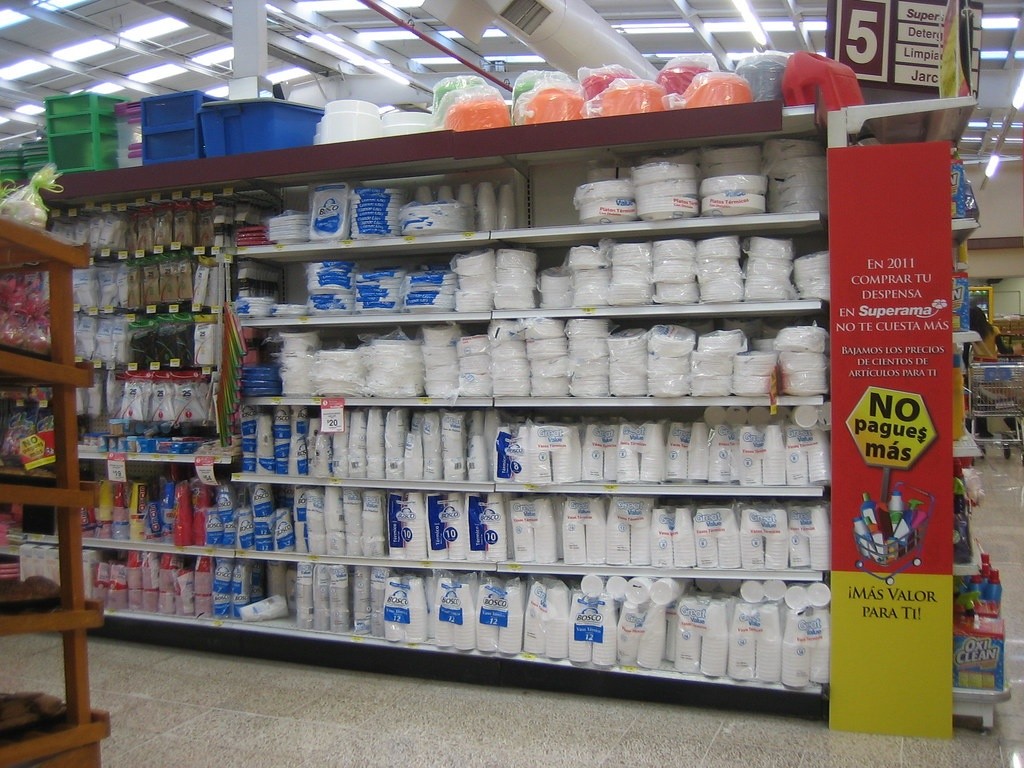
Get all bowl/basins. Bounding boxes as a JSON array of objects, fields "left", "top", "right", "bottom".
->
[{"left": 312, "top": 51, "right": 792, "bottom": 152}]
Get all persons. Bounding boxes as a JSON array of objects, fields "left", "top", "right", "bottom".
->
[{"left": 969, "top": 305, "right": 1024, "bottom": 437}]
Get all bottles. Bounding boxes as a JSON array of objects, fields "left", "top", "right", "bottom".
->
[{"left": 953, "top": 553, "right": 1003, "bottom": 613}]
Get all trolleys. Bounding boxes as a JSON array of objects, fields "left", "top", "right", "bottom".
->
[{"left": 964, "top": 345, "right": 1024, "bottom": 469}]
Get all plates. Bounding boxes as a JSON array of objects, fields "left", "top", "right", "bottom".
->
[{"left": 229, "top": 135, "right": 829, "bottom": 400}]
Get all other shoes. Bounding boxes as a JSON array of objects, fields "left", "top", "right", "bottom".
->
[{"left": 978, "top": 430, "right": 994, "bottom": 438}]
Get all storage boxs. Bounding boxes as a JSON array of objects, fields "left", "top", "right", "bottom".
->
[{"left": 44, "top": 90, "right": 324, "bottom": 175}]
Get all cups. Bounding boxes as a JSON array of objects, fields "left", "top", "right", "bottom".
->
[
  {"left": 203, "top": 407, "right": 833, "bottom": 694},
  {"left": 79, "top": 465, "right": 216, "bottom": 618}
]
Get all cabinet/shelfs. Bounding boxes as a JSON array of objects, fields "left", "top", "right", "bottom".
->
[{"left": 0, "top": 96, "right": 1024, "bottom": 768}]
